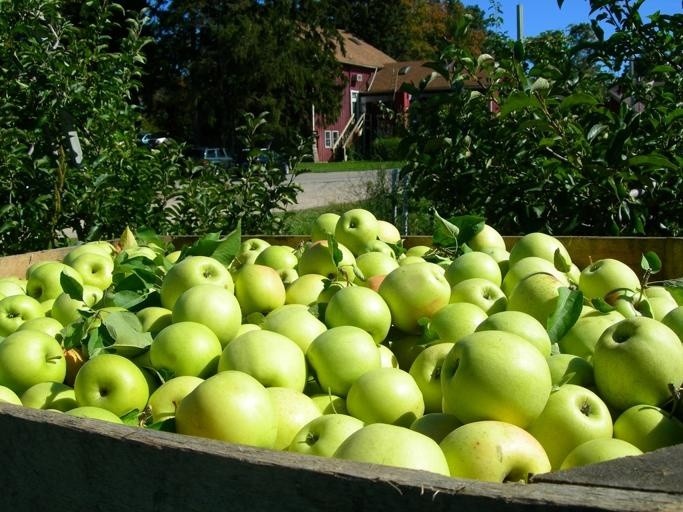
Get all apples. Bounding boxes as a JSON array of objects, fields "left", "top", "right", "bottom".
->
[{"left": 1, "top": 208, "right": 682, "bottom": 482}]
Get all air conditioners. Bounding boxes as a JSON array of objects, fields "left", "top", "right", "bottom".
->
[{"left": 352, "top": 73, "right": 363, "bottom": 82}]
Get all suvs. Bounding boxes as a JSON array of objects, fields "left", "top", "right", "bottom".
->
[
  {"left": 182, "top": 147, "right": 234, "bottom": 176},
  {"left": 238, "top": 144, "right": 292, "bottom": 175}
]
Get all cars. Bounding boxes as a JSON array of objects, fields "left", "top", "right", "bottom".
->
[
  {"left": 131, "top": 131, "right": 151, "bottom": 146},
  {"left": 145, "top": 131, "right": 185, "bottom": 152}
]
